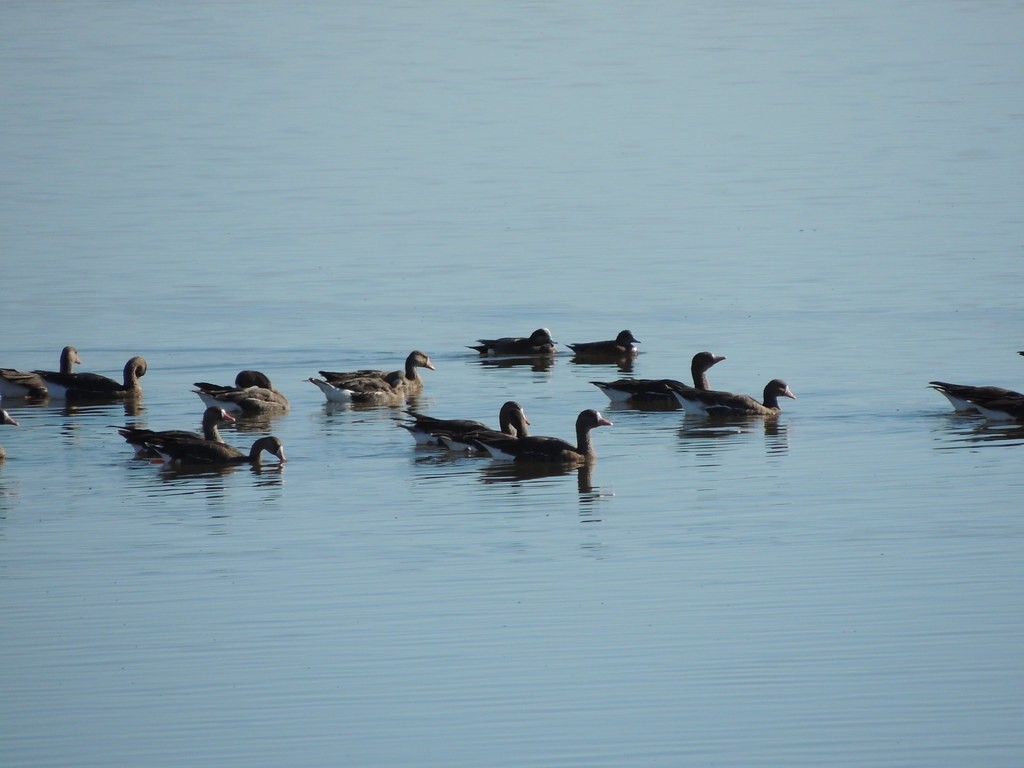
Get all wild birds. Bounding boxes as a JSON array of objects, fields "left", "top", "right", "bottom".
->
[
  {"left": 144, "top": 436, "right": 287, "bottom": 474},
  {"left": 188, "top": 371, "right": 290, "bottom": 422},
  {"left": 470, "top": 409, "right": 613, "bottom": 468},
  {"left": 318, "top": 350, "right": 435, "bottom": 398},
  {"left": 564, "top": 330, "right": 641, "bottom": 357},
  {"left": 300, "top": 370, "right": 409, "bottom": 404},
  {"left": 588, "top": 351, "right": 727, "bottom": 412},
  {"left": 28, "top": 356, "right": 147, "bottom": 401},
  {"left": 105, "top": 406, "right": 236, "bottom": 462},
  {"left": 463, "top": 327, "right": 559, "bottom": 357},
  {"left": 0, "top": 346, "right": 81, "bottom": 397},
  {"left": 431, "top": 409, "right": 529, "bottom": 461},
  {"left": 925, "top": 375, "right": 1024, "bottom": 421},
  {"left": 389, "top": 401, "right": 531, "bottom": 447},
  {"left": 664, "top": 379, "right": 796, "bottom": 417}
]
